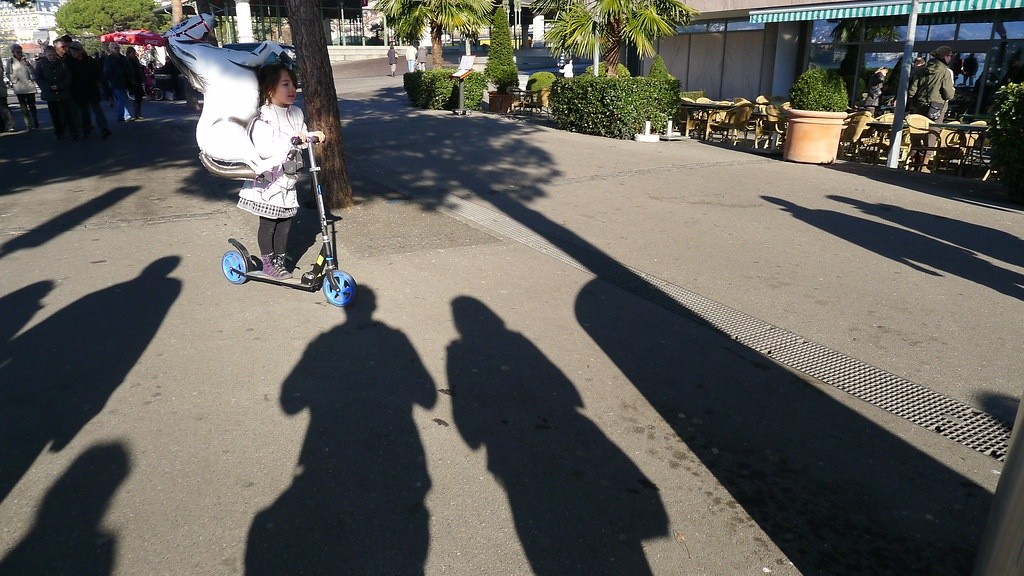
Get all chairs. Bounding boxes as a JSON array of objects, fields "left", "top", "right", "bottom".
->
[
  {"left": 696, "top": 97, "right": 717, "bottom": 139},
  {"left": 954, "top": 86, "right": 968, "bottom": 104},
  {"left": 734, "top": 97, "right": 755, "bottom": 139},
  {"left": 715, "top": 101, "right": 731, "bottom": 122},
  {"left": 954, "top": 120, "right": 987, "bottom": 166},
  {"left": 754, "top": 95, "right": 778, "bottom": 147},
  {"left": 677, "top": 98, "right": 700, "bottom": 137},
  {"left": 902, "top": 114, "right": 956, "bottom": 176},
  {"left": 764, "top": 105, "right": 787, "bottom": 150},
  {"left": 925, "top": 121, "right": 961, "bottom": 165},
  {"left": 704, "top": 103, "right": 756, "bottom": 147},
  {"left": 506, "top": 87, "right": 534, "bottom": 115},
  {"left": 523, "top": 89, "right": 551, "bottom": 116},
  {"left": 898, "top": 114, "right": 911, "bottom": 162},
  {"left": 837, "top": 111, "right": 872, "bottom": 163},
  {"left": 861, "top": 113, "right": 895, "bottom": 156}
]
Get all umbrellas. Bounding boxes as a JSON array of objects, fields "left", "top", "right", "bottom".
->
[{"left": 100, "top": 27, "right": 167, "bottom": 47}]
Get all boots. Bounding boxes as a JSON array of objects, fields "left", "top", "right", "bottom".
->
[
  {"left": 261, "top": 252, "right": 293, "bottom": 280},
  {"left": 909, "top": 153, "right": 931, "bottom": 173}
]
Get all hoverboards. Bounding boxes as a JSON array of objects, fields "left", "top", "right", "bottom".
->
[{"left": 219, "top": 135, "right": 358, "bottom": 309}]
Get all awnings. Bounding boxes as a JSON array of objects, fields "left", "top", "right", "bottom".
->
[{"left": 749, "top": 0, "right": 1024, "bottom": 24}]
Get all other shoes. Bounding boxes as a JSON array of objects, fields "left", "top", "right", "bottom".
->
[
  {"left": 101, "top": 129, "right": 111, "bottom": 138},
  {"left": 85, "top": 128, "right": 92, "bottom": 139}
]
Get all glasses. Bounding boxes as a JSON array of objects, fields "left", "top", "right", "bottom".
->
[
  {"left": 16, "top": 51, "right": 23, "bottom": 54},
  {"left": 947, "top": 55, "right": 953, "bottom": 58},
  {"left": 61, "top": 45, "right": 67, "bottom": 48},
  {"left": 112, "top": 46, "right": 120, "bottom": 50}
]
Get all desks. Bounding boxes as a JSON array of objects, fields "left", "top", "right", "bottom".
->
[
  {"left": 865, "top": 122, "right": 909, "bottom": 164},
  {"left": 931, "top": 123, "right": 991, "bottom": 176},
  {"left": 682, "top": 104, "right": 734, "bottom": 143}
]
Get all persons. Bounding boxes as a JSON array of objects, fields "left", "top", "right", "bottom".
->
[
  {"left": 93, "top": 42, "right": 145, "bottom": 122},
  {"left": 405, "top": 41, "right": 417, "bottom": 73},
  {"left": 387, "top": 44, "right": 398, "bottom": 77},
  {"left": 236, "top": 64, "right": 325, "bottom": 280},
  {"left": 34, "top": 35, "right": 111, "bottom": 141},
  {"left": 144, "top": 65, "right": 152, "bottom": 95},
  {"left": 0, "top": 43, "right": 41, "bottom": 132},
  {"left": 417, "top": 46, "right": 428, "bottom": 71},
  {"left": 962, "top": 53, "right": 978, "bottom": 86},
  {"left": 866, "top": 67, "right": 888, "bottom": 107},
  {"left": 950, "top": 54, "right": 962, "bottom": 82},
  {"left": 907, "top": 46, "right": 956, "bottom": 173}
]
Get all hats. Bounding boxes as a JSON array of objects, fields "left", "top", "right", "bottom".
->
[
  {"left": 930, "top": 46, "right": 950, "bottom": 58},
  {"left": 68, "top": 41, "right": 83, "bottom": 50}
]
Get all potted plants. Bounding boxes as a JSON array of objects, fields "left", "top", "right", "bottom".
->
[
  {"left": 779, "top": 65, "right": 849, "bottom": 166},
  {"left": 525, "top": 71, "right": 557, "bottom": 110},
  {"left": 484, "top": 7, "right": 520, "bottom": 113}
]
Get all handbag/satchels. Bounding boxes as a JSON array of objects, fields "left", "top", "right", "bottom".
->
[
  {"left": 926, "top": 101, "right": 944, "bottom": 120},
  {"left": 394, "top": 54, "right": 398, "bottom": 59},
  {"left": 870, "top": 86, "right": 883, "bottom": 99}
]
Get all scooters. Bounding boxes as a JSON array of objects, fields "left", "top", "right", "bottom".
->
[{"left": 126, "top": 68, "right": 164, "bottom": 101}]
